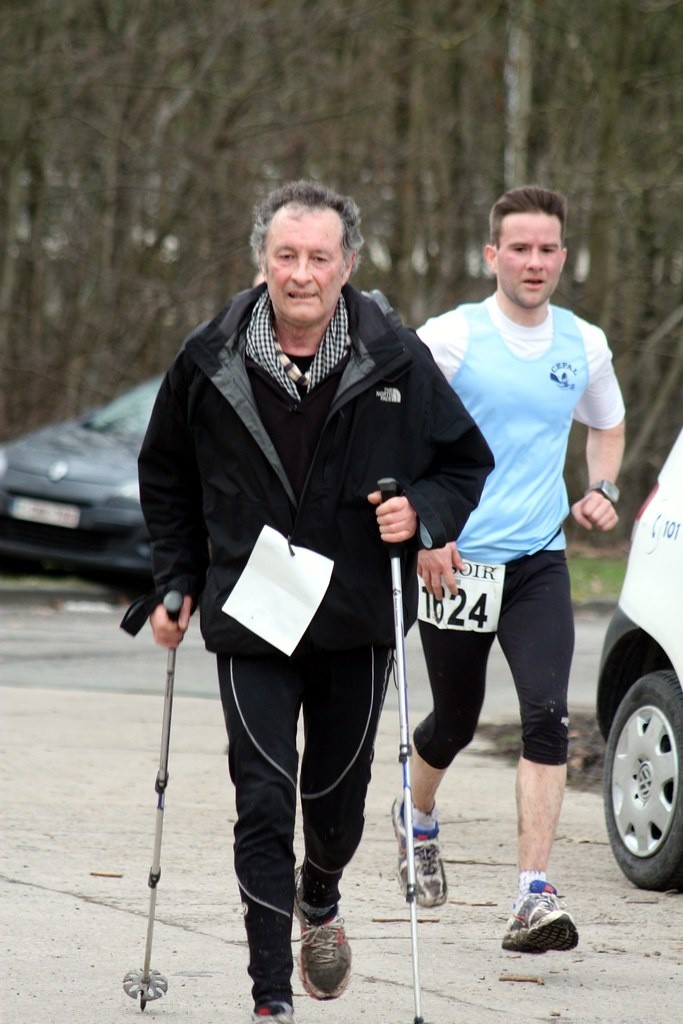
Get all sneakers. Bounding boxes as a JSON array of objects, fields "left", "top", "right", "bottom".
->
[
  {"left": 250, "top": 1001, "right": 293, "bottom": 1024},
  {"left": 292, "top": 865, "right": 351, "bottom": 1000},
  {"left": 391, "top": 796, "right": 447, "bottom": 907},
  {"left": 502, "top": 879, "right": 578, "bottom": 955}
]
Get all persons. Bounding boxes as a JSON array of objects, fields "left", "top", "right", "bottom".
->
[
  {"left": 137, "top": 181, "right": 495, "bottom": 1024},
  {"left": 390, "top": 188, "right": 626, "bottom": 955}
]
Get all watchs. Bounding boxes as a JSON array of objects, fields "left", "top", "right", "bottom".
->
[{"left": 590, "top": 480, "right": 620, "bottom": 503}]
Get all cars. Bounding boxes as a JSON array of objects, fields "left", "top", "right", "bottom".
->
[
  {"left": 1, "top": 370, "right": 170, "bottom": 592},
  {"left": 595, "top": 430, "right": 683, "bottom": 894}
]
